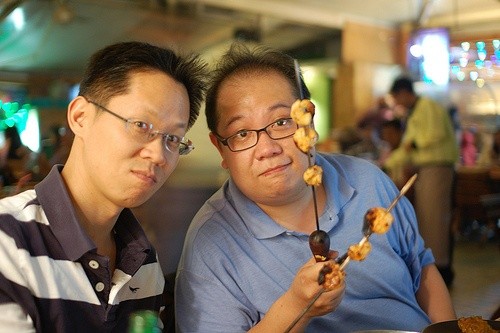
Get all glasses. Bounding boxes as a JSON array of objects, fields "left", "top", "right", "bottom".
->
[
  {"left": 215, "top": 117, "right": 299, "bottom": 153},
  {"left": 89, "top": 99, "right": 195, "bottom": 156}
]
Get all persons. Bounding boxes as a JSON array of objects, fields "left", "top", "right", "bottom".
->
[
  {"left": 173, "top": 39, "right": 458, "bottom": 333},
  {"left": 0, "top": 123, "right": 76, "bottom": 201},
  {"left": 0, "top": 42, "right": 207, "bottom": 333},
  {"left": 339, "top": 76, "right": 500, "bottom": 290}
]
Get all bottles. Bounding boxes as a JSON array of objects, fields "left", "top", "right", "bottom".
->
[{"left": 129, "top": 310, "right": 161, "bottom": 333}]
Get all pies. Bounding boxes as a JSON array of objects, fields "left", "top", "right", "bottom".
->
[{"left": 458, "top": 316, "right": 499, "bottom": 333}]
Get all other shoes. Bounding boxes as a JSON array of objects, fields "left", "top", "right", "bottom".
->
[{"left": 436, "top": 265, "right": 454, "bottom": 290}]
